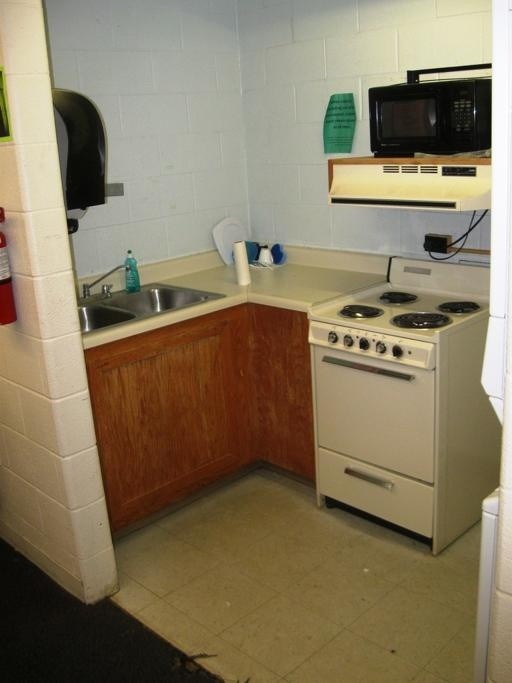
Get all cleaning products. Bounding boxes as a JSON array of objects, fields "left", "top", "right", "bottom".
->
[{"left": 124, "top": 250, "right": 141, "bottom": 294}]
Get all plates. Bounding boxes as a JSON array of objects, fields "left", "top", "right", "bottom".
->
[{"left": 213, "top": 216, "right": 251, "bottom": 267}]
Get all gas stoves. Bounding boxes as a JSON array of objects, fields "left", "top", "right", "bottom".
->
[{"left": 307, "top": 283, "right": 490, "bottom": 370}]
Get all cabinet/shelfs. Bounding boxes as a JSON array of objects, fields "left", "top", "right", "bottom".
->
[
  {"left": 247, "top": 292, "right": 318, "bottom": 486},
  {"left": 82, "top": 302, "right": 248, "bottom": 537}
]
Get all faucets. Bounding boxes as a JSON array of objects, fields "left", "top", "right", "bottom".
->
[{"left": 82, "top": 264, "right": 132, "bottom": 300}]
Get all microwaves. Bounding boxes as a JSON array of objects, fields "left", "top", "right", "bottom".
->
[{"left": 368, "top": 65, "right": 492, "bottom": 158}]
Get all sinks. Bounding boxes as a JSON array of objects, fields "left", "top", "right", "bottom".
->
[
  {"left": 97, "top": 282, "right": 226, "bottom": 318},
  {"left": 76, "top": 282, "right": 134, "bottom": 336}
]
